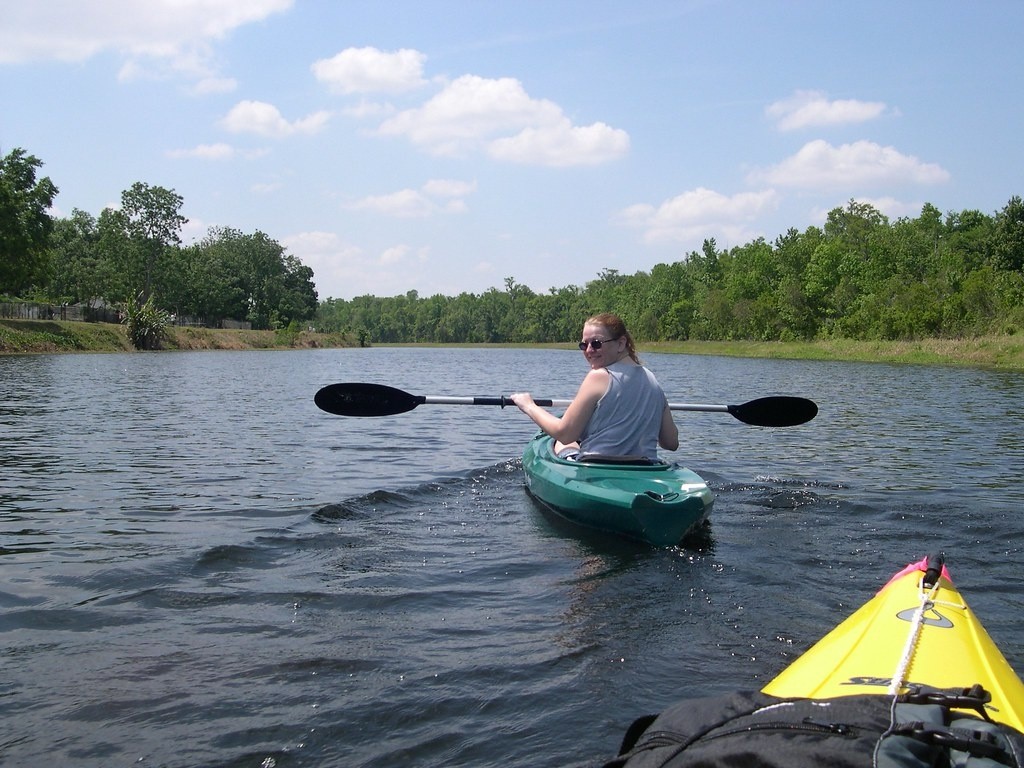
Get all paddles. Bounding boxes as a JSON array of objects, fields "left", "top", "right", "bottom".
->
[{"left": 313, "top": 381, "right": 819, "bottom": 429}]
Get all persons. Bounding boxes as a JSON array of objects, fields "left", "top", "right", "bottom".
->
[
  {"left": 171, "top": 313, "right": 176, "bottom": 326},
  {"left": 511, "top": 314, "right": 679, "bottom": 465}
]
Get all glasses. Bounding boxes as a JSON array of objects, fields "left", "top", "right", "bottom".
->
[{"left": 579, "top": 337, "right": 619, "bottom": 351}]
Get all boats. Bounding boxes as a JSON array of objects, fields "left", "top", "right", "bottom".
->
[
  {"left": 522, "top": 412, "right": 717, "bottom": 549},
  {"left": 761, "top": 553, "right": 1024, "bottom": 738}
]
